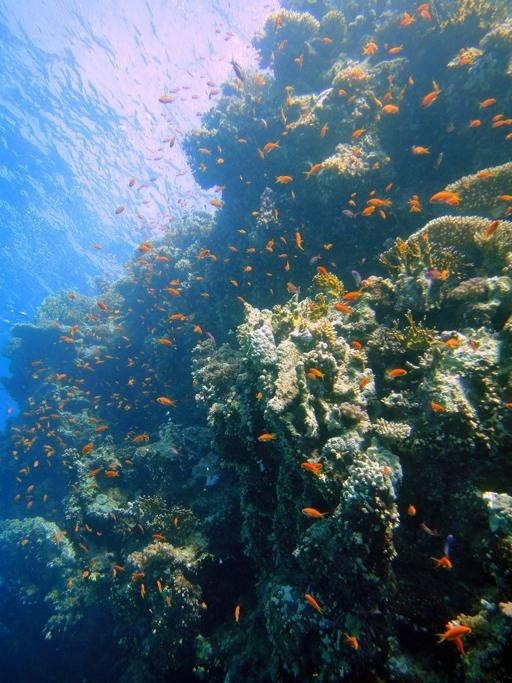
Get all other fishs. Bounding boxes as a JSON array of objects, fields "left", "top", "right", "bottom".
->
[{"left": 6, "top": 0, "right": 511, "bottom": 657}]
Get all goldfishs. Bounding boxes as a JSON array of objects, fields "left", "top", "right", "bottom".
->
[{"left": 6, "top": 0, "right": 511, "bottom": 657}]
